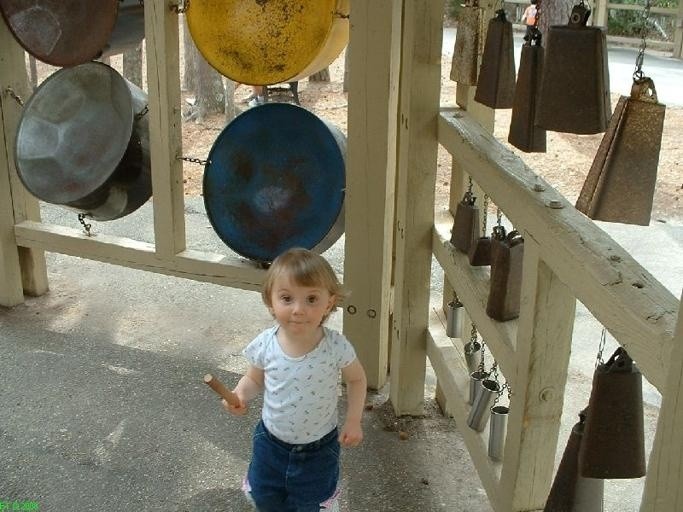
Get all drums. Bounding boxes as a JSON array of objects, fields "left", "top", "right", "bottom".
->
[
  {"left": 1, "top": 1, "right": 144, "bottom": 66},
  {"left": 13, "top": 62, "right": 152, "bottom": 221},
  {"left": 185, "top": 0, "right": 350, "bottom": 85},
  {"left": 202, "top": 102, "right": 345, "bottom": 264}
]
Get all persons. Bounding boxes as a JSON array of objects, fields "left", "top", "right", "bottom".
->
[
  {"left": 220, "top": 249, "right": 367, "bottom": 509},
  {"left": 520, "top": 1, "right": 539, "bottom": 40}
]
